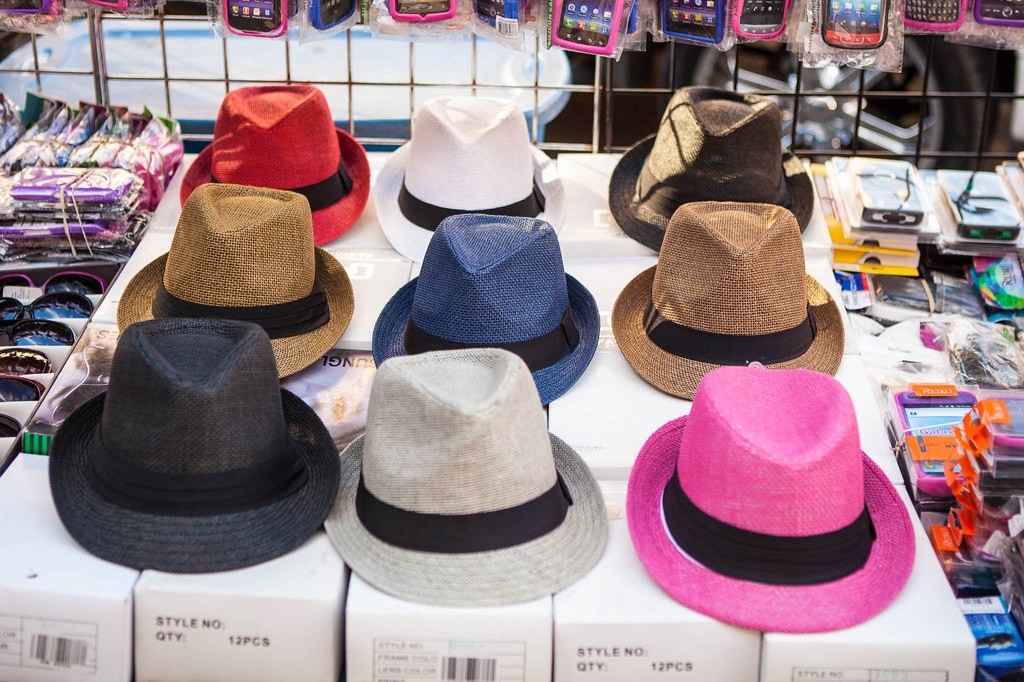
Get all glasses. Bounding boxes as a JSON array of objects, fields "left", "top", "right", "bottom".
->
[
  {"left": 0, "top": 348, "right": 52, "bottom": 374},
  {"left": 0, "top": 321, "right": 78, "bottom": 347},
  {"left": 0, "top": 271, "right": 107, "bottom": 295},
  {"left": 0, "top": 292, "right": 94, "bottom": 319},
  {"left": 0, "top": 374, "right": 46, "bottom": 403}
]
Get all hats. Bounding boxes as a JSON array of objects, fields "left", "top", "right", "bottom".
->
[
  {"left": 372, "top": 213, "right": 601, "bottom": 406},
  {"left": 180, "top": 85, "right": 370, "bottom": 246},
  {"left": 626, "top": 366, "right": 915, "bottom": 633},
  {"left": 609, "top": 86, "right": 814, "bottom": 254},
  {"left": 324, "top": 348, "right": 609, "bottom": 607},
  {"left": 374, "top": 97, "right": 565, "bottom": 265},
  {"left": 48, "top": 317, "right": 340, "bottom": 573},
  {"left": 116, "top": 183, "right": 355, "bottom": 379},
  {"left": 611, "top": 201, "right": 845, "bottom": 398}
]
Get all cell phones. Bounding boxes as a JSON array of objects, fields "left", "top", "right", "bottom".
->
[{"left": 0, "top": 0, "right": 1024, "bottom": 682}]
